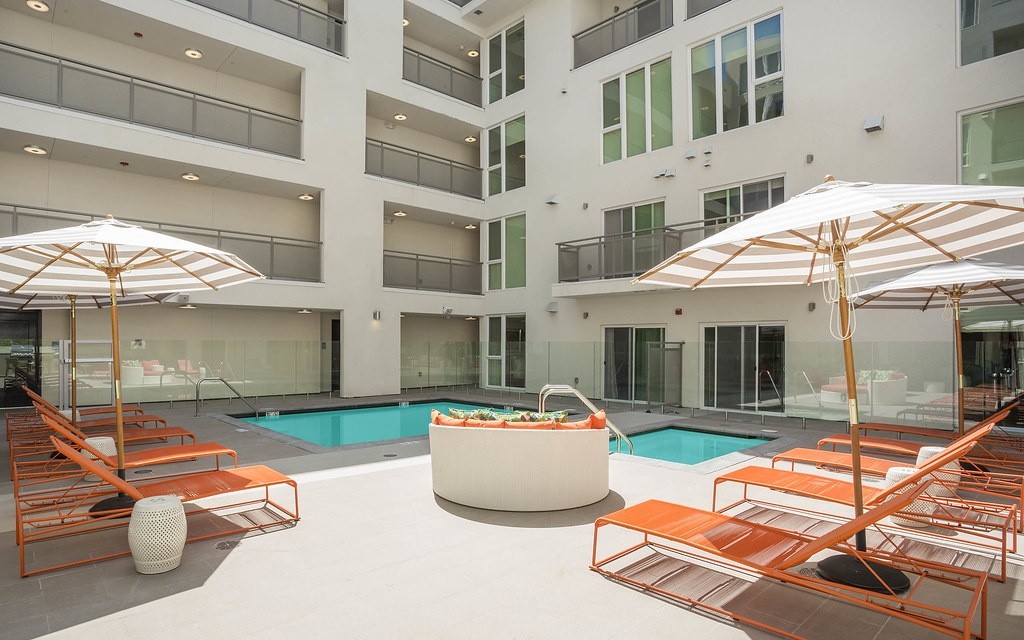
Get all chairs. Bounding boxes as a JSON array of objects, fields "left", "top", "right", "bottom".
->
[
  {"left": 178, "top": 360, "right": 199, "bottom": 375},
  {"left": 3, "top": 363, "right": 94, "bottom": 406},
  {"left": 7, "top": 385, "right": 300, "bottom": 578},
  {"left": 897, "top": 383, "right": 1024, "bottom": 428},
  {"left": 589, "top": 401, "right": 1024, "bottom": 640}
]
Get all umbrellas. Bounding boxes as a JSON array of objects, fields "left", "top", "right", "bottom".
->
[
  {"left": 0, "top": 215, "right": 267, "bottom": 522},
  {"left": 630, "top": 175, "right": 1024, "bottom": 599}
]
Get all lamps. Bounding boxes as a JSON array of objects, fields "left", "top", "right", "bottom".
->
[
  {"left": 180, "top": 304, "right": 197, "bottom": 309},
  {"left": 299, "top": 193, "right": 314, "bottom": 200},
  {"left": 297, "top": 309, "right": 314, "bottom": 313},
  {"left": 23, "top": 144, "right": 200, "bottom": 182},
  {"left": 393, "top": 113, "right": 477, "bottom": 143},
  {"left": 373, "top": 311, "right": 477, "bottom": 321},
  {"left": 394, "top": 211, "right": 476, "bottom": 230},
  {"left": 22, "top": 0, "right": 202, "bottom": 60},
  {"left": 394, "top": 17, "right": 479, "bottom": 59}
]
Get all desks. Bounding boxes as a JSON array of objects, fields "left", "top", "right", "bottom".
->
[
  {"left": 127, "top": 495, "right": 187, "bottom": 575},
  {"left": 917, "top": 446, "right": 962, "bottom": 497},
  {"left": 885, "top": 467, "right": 936, "bottom": 527},
  {"left": 56, "top": 409, "right": 82, "bottom": 438},
  {"left": 80, "top": 436, "right": 117, "bottom": 482}
]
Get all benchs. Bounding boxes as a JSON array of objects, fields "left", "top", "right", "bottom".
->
[
  {"left": 820, "top": 372, "right": 907, "bottom": 405},
  {"left": 107, "top": 359, "right": 175, "bottom": 386},
  {"left": 429, "top": 408, "right": 610, "bottom": 512}
]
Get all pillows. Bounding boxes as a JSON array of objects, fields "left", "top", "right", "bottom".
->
[
  {"left": 857, "top": 369, "right": 892, "bottom": 385},
  {"left": 449, "top": 408, "right": 569, "bottom": 423}
]
